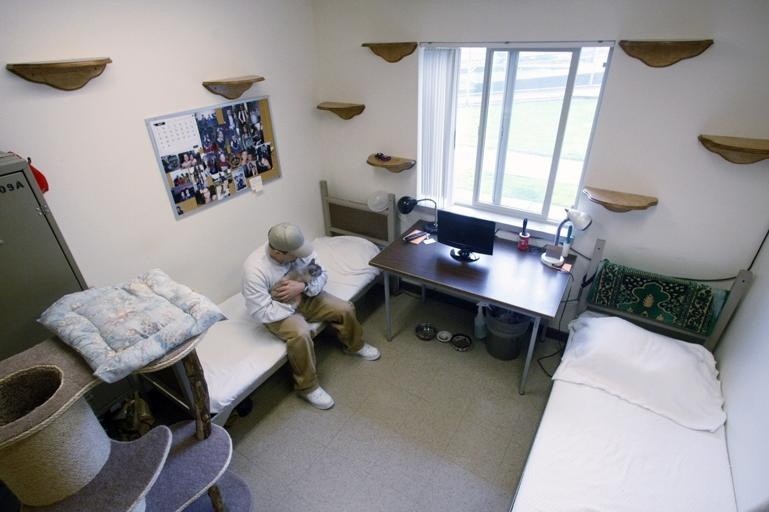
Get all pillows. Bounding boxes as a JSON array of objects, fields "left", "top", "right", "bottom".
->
[{"left": 551, "top": 316, "right": 727, "bottom": 432}]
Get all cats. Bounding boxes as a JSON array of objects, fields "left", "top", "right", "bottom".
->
[{"left": 268, "top": 258, "right": 322, "bottom": 304}]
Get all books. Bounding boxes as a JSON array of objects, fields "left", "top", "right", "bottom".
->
[{"left": 402, "top": 229, "right": 431, "bottom": 245}]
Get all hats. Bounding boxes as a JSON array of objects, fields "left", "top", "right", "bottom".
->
[{"left": 268, "top": 223, "right": 315, "bottom": 258}]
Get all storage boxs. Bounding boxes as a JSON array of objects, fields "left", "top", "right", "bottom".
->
[{"left": 313, "top": 337, "right": 325, "bottom": 365}]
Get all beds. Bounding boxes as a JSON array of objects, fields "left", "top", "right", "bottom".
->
[
  {"left": 509, "top": 240, "right": 752, "bottom": 512},
  {"left": 168, "top": 179, "right": 396, "bottom": 427}
]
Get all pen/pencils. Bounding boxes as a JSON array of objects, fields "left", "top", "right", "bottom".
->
[{"left": 522, "top": 218, "right": 529, "bottom": 236}]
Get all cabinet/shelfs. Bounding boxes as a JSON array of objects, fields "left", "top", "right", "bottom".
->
[
  {"left": 0, "top": 324, "right": 253, "bottom": 512},
  {"left": 0, "top": 150, "right": 90, "bottom": 362}
]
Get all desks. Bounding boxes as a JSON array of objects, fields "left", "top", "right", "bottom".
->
[{"left": 368, "top": 218, "right": 577, "bottom": 395}]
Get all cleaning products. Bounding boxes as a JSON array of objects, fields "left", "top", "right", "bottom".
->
[{"left": 474, "top": 302, "right": 495, "bottom": 338}]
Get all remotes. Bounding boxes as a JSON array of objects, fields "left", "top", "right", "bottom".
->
[{"left": 403, "top": 230, "right": 427, "bottom": 243}]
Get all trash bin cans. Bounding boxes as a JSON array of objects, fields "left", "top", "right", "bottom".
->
[{"left": 482, "top": 305, "right": 532, "bottom": 361}]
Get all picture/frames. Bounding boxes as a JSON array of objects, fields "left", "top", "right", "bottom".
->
[{"left": 144, "top": 96, "right": 281, "bottom": 221}]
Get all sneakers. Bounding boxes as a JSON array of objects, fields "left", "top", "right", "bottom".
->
[
  {"left": 301, "top": 386, "right": 334, "bottom": 409},
  {"left": 343, "top": 342, "right": 381, "bottom": 361}
]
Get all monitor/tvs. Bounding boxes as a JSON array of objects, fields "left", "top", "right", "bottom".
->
[{"left": 435, "top": 208, "right": 496, "bottom": 263}]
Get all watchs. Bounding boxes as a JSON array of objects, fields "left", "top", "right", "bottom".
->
[{"left": 302, "top": 282, "right": 310, "bottom": 294}]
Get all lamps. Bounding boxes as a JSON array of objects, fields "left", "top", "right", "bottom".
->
[
  {"left": 398, "top": 195, "right": 438, "bottom": 234},
  {"left": 541, "top": 207, "right": 592, "bottom": 269}
]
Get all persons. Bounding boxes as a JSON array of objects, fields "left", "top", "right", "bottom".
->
[
  {"left": 241, "top": 222, "right": 380, "bottom": 413},
  {"left": 161, "top": 102, "right": 273, "bottom": 207}
]
